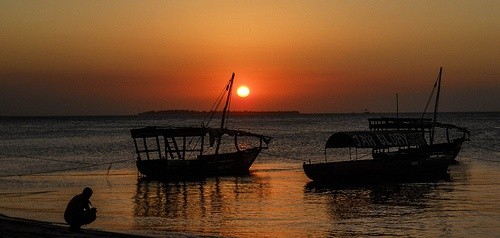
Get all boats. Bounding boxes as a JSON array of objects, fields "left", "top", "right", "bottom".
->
[
  {"left": 368, "top": 118, "right": 432, "bottom": 130},
  {"left": 130, "top": 72, "right": 271, "bottom": 180},
  {"left": 302, "top": 129, "right": 466, "bottom": 185}
]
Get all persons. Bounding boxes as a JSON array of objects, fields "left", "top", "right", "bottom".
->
[{"left": 63, "top": 186, "right": 98, "bottom": 234}]
